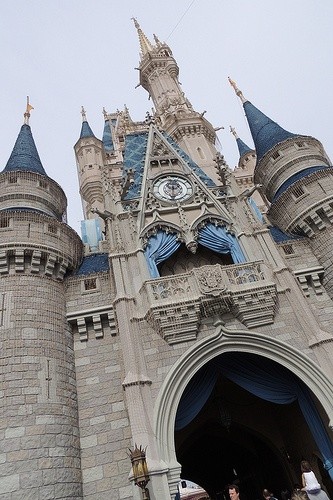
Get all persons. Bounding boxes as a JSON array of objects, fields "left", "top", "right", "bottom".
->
[
  {"left": 226, "top": 484, "right": 242, "bottom": 500},
  {"left": 262, "top": 488, "right": 278, "bottom": 500},
  {"left": 299, "top": 461, "right": 330, "bottom": 500}
]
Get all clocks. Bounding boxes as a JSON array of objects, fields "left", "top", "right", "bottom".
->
[{"left": 151, "top": 173, "right": 193, "bottom": 206}]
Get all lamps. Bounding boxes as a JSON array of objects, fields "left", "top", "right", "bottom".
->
[{"left": 125, "top": 443, "right": 149, "bottom": 484}]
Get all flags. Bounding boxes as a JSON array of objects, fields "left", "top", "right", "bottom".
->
[{"left": 27, "top": 104, "right": 36, "bottom": 113}]
